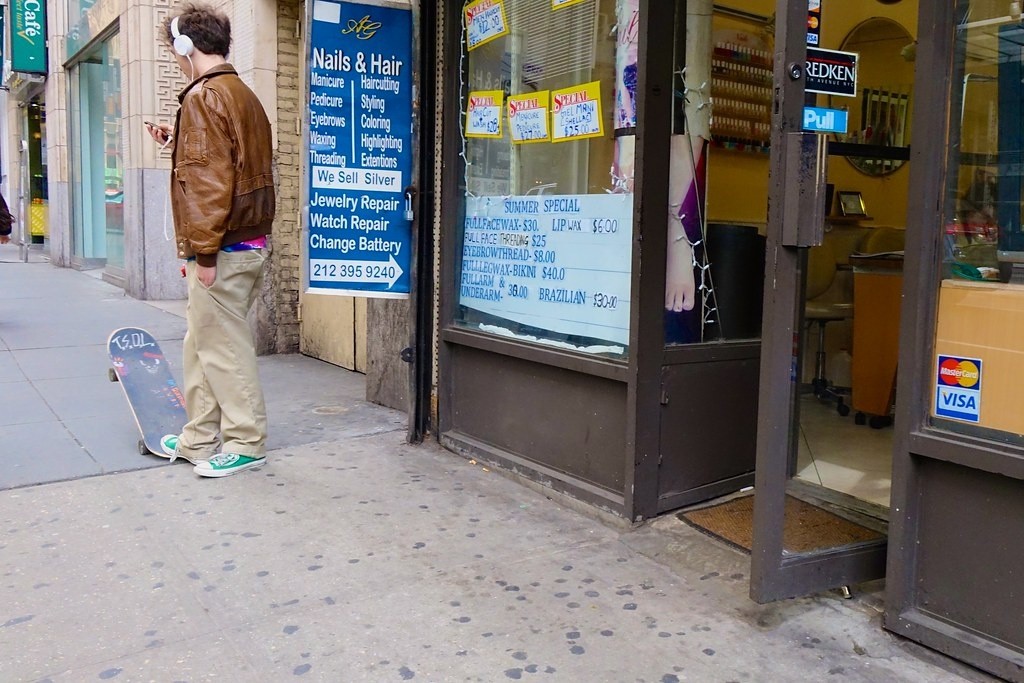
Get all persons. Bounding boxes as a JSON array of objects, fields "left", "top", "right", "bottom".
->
[
  {"left": 944, "top": 181, "right": 1015, "bottom": 281},
  {"left": 0, "top": 193, "right": 12, "bottom": 244},
  {"left": 616, "top": 0, "right": 711, "bottom": 312},
  {"left": 145, "top": 3, "right": 275, "bottom": 478}
]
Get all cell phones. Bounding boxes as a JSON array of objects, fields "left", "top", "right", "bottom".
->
[{"left": 144, "top": 121, "right": 169, "bottom": 141}]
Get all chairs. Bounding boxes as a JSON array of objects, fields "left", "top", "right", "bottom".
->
[{"left": 805, "top": 240, "right": 854, "bottom": 417}]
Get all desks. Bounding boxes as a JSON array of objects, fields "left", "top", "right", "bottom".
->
[{"left": 849, "top": 253, "right": 902, "bottom": 429}]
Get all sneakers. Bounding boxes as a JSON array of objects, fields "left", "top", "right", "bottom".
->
[
  {"left": 159, "top": 433, "right": 198, "bottom": 467},
  {"left": 193, "top": 452, "right": 268, "bottom": 478}
]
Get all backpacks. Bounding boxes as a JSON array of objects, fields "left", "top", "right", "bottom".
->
[{"left": 0, "top": 188, "right": 17, "bottom": 236}]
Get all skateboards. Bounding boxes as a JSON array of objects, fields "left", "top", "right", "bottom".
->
[{"left": 108, "top": 327, "right": 198, "bottom": 458}]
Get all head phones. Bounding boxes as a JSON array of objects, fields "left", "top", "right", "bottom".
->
[{"left": 171, "top": 16, "right": 194, "bottom": 56}]
